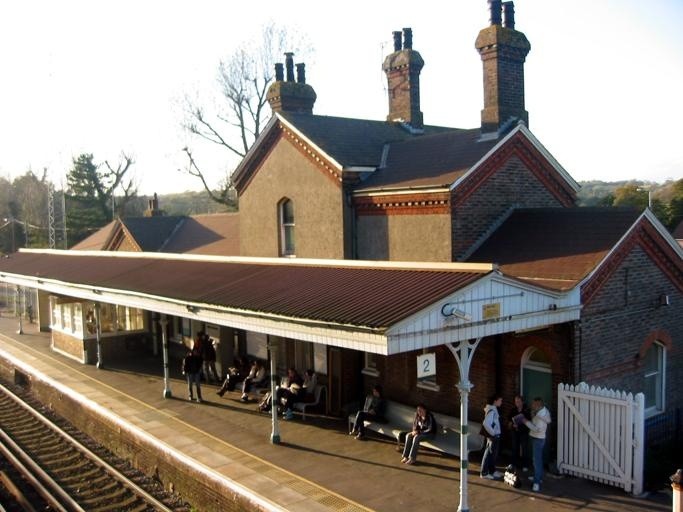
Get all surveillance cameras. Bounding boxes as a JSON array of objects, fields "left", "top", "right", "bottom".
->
[{"left": 451, "top": 308, "right": 472, "bottom": 321}]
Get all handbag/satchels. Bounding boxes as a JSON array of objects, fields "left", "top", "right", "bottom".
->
[
  {"left": 503, "top": 463, "right": 522, "bottom": 489},
  {"left": 479, "top": 409, "right": 496, "bottom": 438}
]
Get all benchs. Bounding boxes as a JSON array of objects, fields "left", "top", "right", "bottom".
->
[
  {"left": 280, "top": 376, "right": 328, "bottom": 420},
  {"left": 348, "top": 399, "right": 416, "bottom": 452},
  {"left": 419, "top": 411, "right": 486, "bottom": 462},
  {"left": 236, "top": 371, "right": 279, "bottom": 396}
]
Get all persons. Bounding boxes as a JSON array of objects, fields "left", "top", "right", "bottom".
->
[
  {"left": 180, "top": 351, "right": 203, "bottom": 404},
  {"left": 520, "top": 396, "right": 552, "bottom": 492},
  {"left": 192, "top": 331, "right": 317, "bottom": 421},
  {"left": 399, "top": 404, "right": 438, "bottom": 465},
  {"left": 506, "top": 394, "right": 532, "bottom": 473},
  {"left": 348, "top": 385, "right": 389, "bottom": 440},
  {"left": 478, "top": 393, "right": 505, "bottom": 479}
]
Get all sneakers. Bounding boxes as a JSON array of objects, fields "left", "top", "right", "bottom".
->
[
  {"left": 188, "top": 396, "right": 203, "bottom": 403},
  {"left": 528, "top": 476, "right": 543, "bottom": 492},
  {"left": 349, "top": 429, "right": 365, "bottom": 440},
  {"left": 479, "top": 470, "right": 502, "bottom": 480},
  {"left": 400, "top": 458, "right": 416, "bottom": 464}
]
